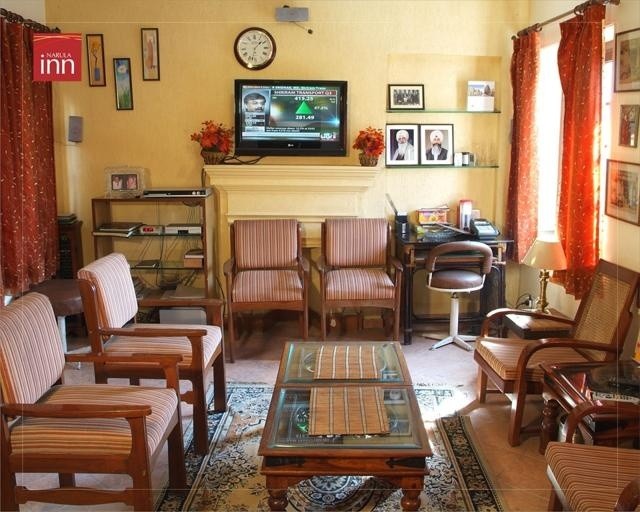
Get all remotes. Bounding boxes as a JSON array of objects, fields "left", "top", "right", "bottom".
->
[{"left": 592, "top": 392, "right": 640, "bottom": 406}]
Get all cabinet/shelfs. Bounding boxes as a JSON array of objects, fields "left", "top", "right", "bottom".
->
[
  {"left": 384, "top": 110, "right": 501, "bottom": 169},
  {"left": 90, "top": 191, "right": 218, "bottom": 326}
]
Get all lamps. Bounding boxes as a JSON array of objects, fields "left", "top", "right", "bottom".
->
[{"left": 521, "top": 238, "right": 567, "bottom": 315}]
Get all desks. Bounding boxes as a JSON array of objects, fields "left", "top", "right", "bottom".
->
[{"left": 390, "top": 225, "right": 513, "bottom": 345}]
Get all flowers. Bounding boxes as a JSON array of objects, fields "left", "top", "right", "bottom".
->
[
  {"left": 351, "top": 128, "right": 387, "bottom": 158},
  {"left": 189, "top": 120, "right": 235, "bottom": 156}
]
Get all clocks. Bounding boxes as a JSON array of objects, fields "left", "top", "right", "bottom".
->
[{"left": 234, "top": 28, "right": 277, "bottom": 72}]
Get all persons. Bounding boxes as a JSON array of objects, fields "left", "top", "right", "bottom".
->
[
  {"left": 125, "top": 175, "right": 136, "bottom": 190},
  {"left": 113, "top": 175, "right": 123, "bottom": 190},
  {"left": 242, "top": 93, "right": 268, "bottom": 131},
  {"left": 395, "top": 88, "right": 421, "bottom": 106},
  {"left": 426, "top": 129, "right": 448, "bottom": 163},
  {"left": 390, "top": 130, "right": 412, "bottom": 161}
]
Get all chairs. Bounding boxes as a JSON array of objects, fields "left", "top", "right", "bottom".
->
[
  {"left": 220, "top": 217, "right": 405, "bottom": 364},
  {"left": 420, "top": 239, "right": 494, "bottom": 352}
]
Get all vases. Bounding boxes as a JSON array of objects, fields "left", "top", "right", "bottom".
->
[
  {"left": 201, "top": 149, "right": 227, "bottom": 165},
  {"left": 359, "top": 152, "right": 378, "bottom": 166}
]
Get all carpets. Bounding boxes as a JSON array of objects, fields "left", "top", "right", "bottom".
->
[{"left": 155, "top": 380, "right": 505, "bottom": 512}]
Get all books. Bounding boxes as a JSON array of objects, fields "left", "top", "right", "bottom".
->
[
  {"left": 184, "top": 247, "right": 203, "bottom": 259},
  {"left": 136, "top": 258, "right": 158, "bottom": 269},
  {"left": 100, "top": 220, "right": 140, "bottom": 231},
  {"left": 92, "top": 223, "right": 147, "bottom": 238}
]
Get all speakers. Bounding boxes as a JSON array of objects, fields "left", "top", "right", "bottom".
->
[{"left": 274, "top": 8, "right": 310, "bottom": 22}]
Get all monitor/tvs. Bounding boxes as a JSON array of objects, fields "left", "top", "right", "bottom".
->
[{"left": 235, "top": 79, "right": 347, "bottom": 156}]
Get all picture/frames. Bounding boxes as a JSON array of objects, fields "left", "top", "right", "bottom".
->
[
  {"left": 85, "top": 27, "right": 160, "bottom": 112},
  {"left": 388, "top": 83, "right": 425, "bottom": 111},
  {"left": 384, "top": 123, "right": 454, "bottom": 167},
  {"left": 104, "top": 166, "right": 146, "bottom": 199},
  {"left": 604, "top": 28, "right": 639, "bottom": 227}
]
